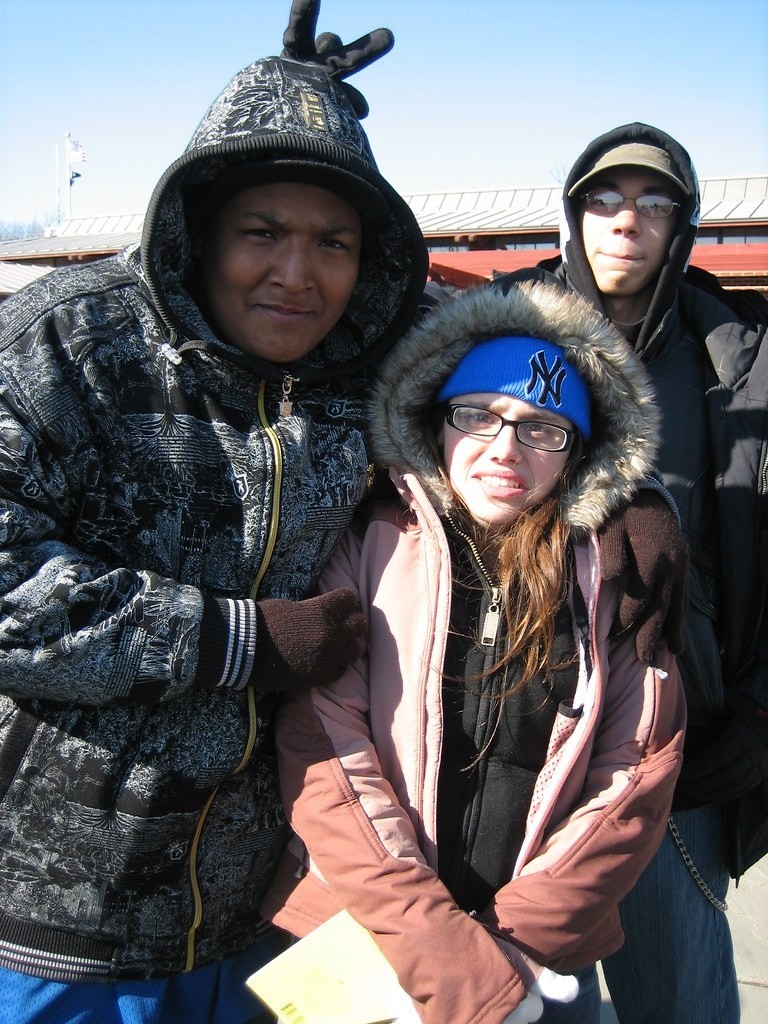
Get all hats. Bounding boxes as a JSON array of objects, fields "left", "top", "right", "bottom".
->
[
  {"left": 438, "top": 336, "right": 592, "bottom": 440},
  {"left": 567, "top": 139, "right": 690, "bottom": 198}
]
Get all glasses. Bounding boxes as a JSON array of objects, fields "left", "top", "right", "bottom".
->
[
  {"left": 443, "top": 404, "right": 575, "bottom": 452},
  {"left": 578, "top": 188, "right": 680, "bottom": 218}
]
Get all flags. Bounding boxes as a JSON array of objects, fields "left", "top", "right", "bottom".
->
[{"left": 68, "top": 141, "right": 85, "bottom": 162}]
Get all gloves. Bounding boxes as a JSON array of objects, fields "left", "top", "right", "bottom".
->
[
  {"left": 599, "top": 489, "right": 689, "bottom": 665},
  {"left": 501, "top": 965, "right": 580, "bottom": 1024},
  {"left": 281, "top": 0, "right": 395, "bottom": 121},
  {"left": 246, "top": 587, "right": 368, "bottom": 686}
]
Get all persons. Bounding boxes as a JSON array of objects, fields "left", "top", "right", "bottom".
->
[
  {"left": 231, "top": 274, "right": 690, "bottom": 1022},
  {"left": 2, "top": 56, "right": 689, "bottom": 1021},
  {"left": 497, "top": 122, "right": 767, "bottom": 1024}
]
